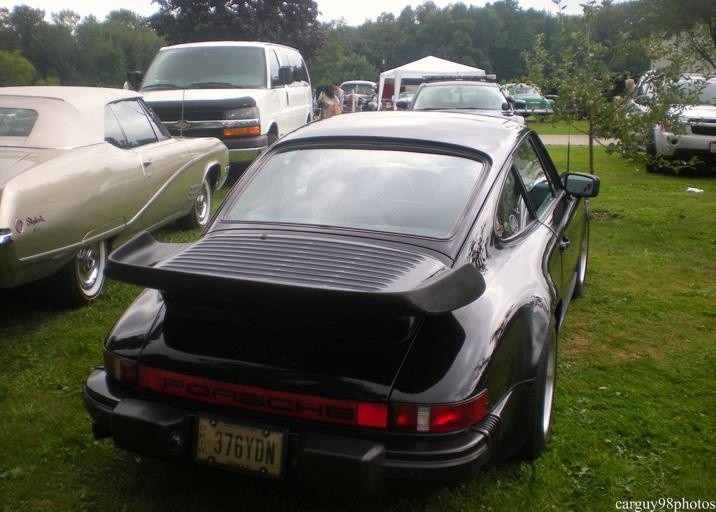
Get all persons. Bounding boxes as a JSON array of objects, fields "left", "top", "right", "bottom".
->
[{"left": 316, "top": 78, "right": 345, "bottom": 121}]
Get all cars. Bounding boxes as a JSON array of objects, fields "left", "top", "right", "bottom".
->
[
  {"left": 0, "top": 40, "right": 600, "bottom": 492},
  {"left": 622, "top": 70, "right": 716, "bottom": 172}
]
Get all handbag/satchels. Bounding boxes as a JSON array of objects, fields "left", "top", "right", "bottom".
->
[
  {"left": 334, "top": 104, "right": 341, "bottom": 114},
  {"left": 318, "top": 92, "right": 325, "bottom": 108}
]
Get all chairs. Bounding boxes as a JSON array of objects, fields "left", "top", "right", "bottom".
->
[{"left": 286, "top": 166, "right": 442, "bottom": 225}]
